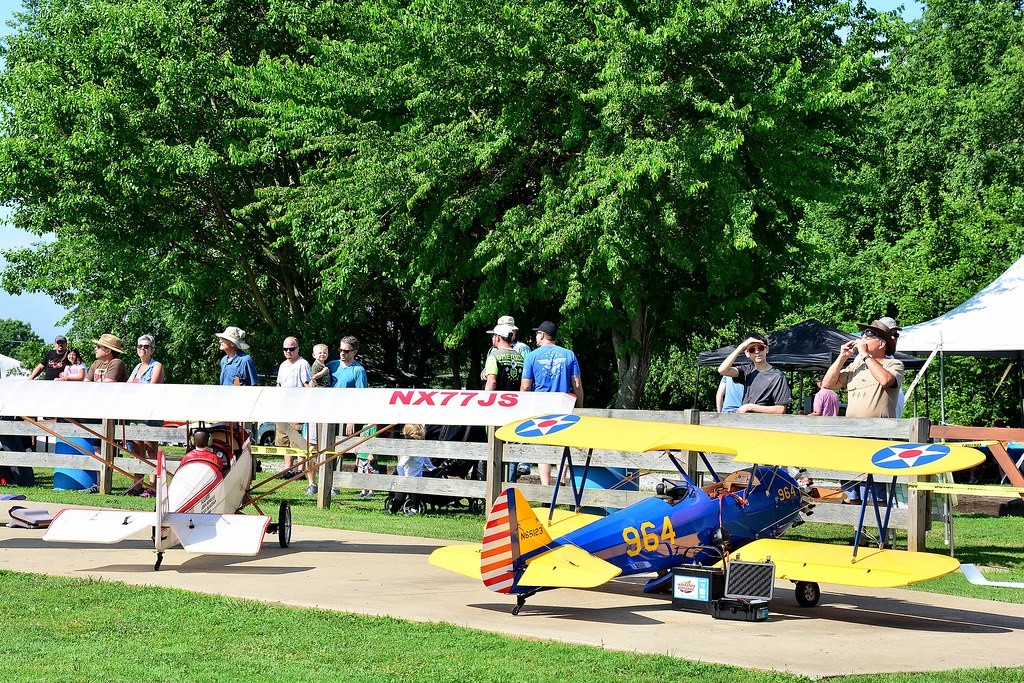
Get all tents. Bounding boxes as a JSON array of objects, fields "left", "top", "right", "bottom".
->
[
  {"left": 695, "top": 319, "right": 930, "bottom": 418},
  {"left": 853, "top": 256, "right": 1024, "bottom": 556}
]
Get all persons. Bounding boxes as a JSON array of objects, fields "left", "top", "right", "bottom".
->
[
  {"left": 311, "top": 344, "right": 331, "bottom": 389},
  {"left": 520, "top": 322, "right": 583, "bottom": 508},
  {"left": 716, "top": 376, "right": 744, "bottom": 412},
  {"left": 274, "top": 336, "right": 310, "bottom": 478},
  {"left": 808, "top": 375, "right": 839, "bottom": 415},
  {"left": 85, "top": 334, "right": 126, "bottom": 382},
  {"left": 304, "top": 337, "right": 367, "bottom": 494},
  {"left": 356, "top": 424, "right": 377, "bottom": 497},
  {"left": 479, "top": 315, "right": 530, "bottom": 483},
  {"left": 120, "top": 334, "right": 165, "bottom": 499},
  {"left": 384, "top": 424, "right": 437, "bottom": 517},
  {"left": 28, "top": 335, "right": 87, "bottom": 420},
  {"left": 216, "top": 326, "right": 257, "bottom": 385},
  {"left": 180, "top": 429, "right": 223, "bottom": 469},
  {"left": 821, "top": 317, "right": 904, "bottom": 506},
  {"left": 717, "top": 332, "right": 792, "bottom": 415}
]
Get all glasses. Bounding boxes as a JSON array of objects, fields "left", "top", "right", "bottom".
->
[
  {"left": 97, "top": 345, "right": 108, "bottom": 350},
  {"left": 338, "top": 347, "right": 357, "bottom": 354},
  {"left": 136, "top": 344, "right": 153, "bottom": 350},
  {"left": 746, "top": 344, "right": 767, "bottom": 354},
  {"left": 535, "top": 331, "right": 547, "bottom": 336},
  {"left": 891, "top": 332, "right": 900, "bottom": 338},
  {"left": 56, "top": 341, "right": 65, "bottom": 345},
  {"left": 283, "top": 347, "right": 298, "bottom": 352},
  {"left": 860, "top": 330, "right": 884, "bottom": 341}
]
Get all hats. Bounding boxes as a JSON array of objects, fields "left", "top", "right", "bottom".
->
[
  {"left": 857, "top": 320, "right": 896, "bottom": 356},
  {"left": 879, "top": 317, "right": 902, "bottom": 331},
  {"left": 486, "top": 324, "right": 514, "bottom": 338},
  {"left": 91, "top": 334, "right": 125, "bottom": 353},
  {"left": 498, "top": 315, "right": 519, "bottom": 331},
  {"left": 215, "top": 326, "right": 250, "bottom": 350},
  {"left": 54, "top": 335, "right": 67, "bottom": 344},
  {"left": 532, "top": 321, "right": 557, "bottom": 338}
]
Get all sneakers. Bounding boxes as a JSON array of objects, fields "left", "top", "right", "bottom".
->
[{"left": 78, "top": 483, "right": 101, "bottom": 495}]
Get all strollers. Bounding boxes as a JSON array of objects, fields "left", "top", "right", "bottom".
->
[{"left": 384, "top": 425, "right": 485, "bottom": 514}]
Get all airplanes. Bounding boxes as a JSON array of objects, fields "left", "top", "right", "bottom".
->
[
  {"left": 430, "top": 414, "right": 987, "bottom": 620},
  {"left": 0, "top": 377, "right": 579, "bottom": 571}
]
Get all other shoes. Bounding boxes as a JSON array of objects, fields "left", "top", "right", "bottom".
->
[
  {"left": 331, "top": 487, "right": 341, "bottom": 498},
  {"left": 305, "top": 483, "right": 318, "bottom": 496},
  {"left": 126, "top": 485, "right": 156, "bottom": 498},
  {"left": 355, "top": 491, "right": 374, "bottom": 498},
  {"left": 542, "top": 502, "right": 552, "bottom": 508},
  {"left": 276, "top": 470, "right": 307, "bottom": 480}
]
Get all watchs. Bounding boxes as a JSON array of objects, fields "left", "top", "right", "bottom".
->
[{"left": 863, "top": 353, "right": 873, "bottom": 361}]
